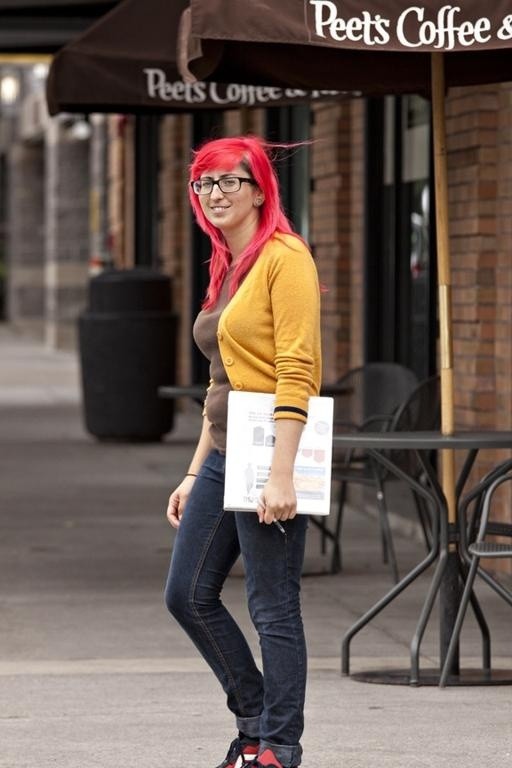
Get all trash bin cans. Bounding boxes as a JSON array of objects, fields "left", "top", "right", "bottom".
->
[{"left": 74, "top": 271, "right": 180, "bottom": 443}]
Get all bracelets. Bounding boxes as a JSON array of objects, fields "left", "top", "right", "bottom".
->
[{"left": 185, "top": 472, "right": 199, "bottom": 478}]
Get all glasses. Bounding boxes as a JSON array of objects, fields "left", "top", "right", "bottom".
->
[{"left": 190, "top": 177, "right": 253, "bottom": 194}]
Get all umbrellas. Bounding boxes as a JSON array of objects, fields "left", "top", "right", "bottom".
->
[{"left": 41, "top": 0, "right": 512, "bottom": 674}]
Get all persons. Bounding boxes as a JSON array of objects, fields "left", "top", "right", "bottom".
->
[{"left": 167, "top": 136, "right": 325, "bottom": 767}]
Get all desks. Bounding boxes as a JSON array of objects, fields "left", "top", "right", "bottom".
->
[
  {"left": 330, "top": 428, "right": 510, "bottom": 690},
  {"left": 157, "top": 378, "right": 353, "bottom": 575}
]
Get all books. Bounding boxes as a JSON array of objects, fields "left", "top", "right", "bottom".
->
[{"left": 221, "top": 389, "right": 336, "bottom": 516}]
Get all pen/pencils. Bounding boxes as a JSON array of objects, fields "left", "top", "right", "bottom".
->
[{"left": 257, "top": 498, "right": 288, "bottom": 537}]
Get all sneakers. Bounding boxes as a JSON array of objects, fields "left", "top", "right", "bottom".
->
[{"left": 217, "top": 736, "right": 281, "bottom": 768}]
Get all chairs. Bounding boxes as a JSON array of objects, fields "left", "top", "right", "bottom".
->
[{"left": 319, "top": 360, "right": 443, "bottom": 591}]
